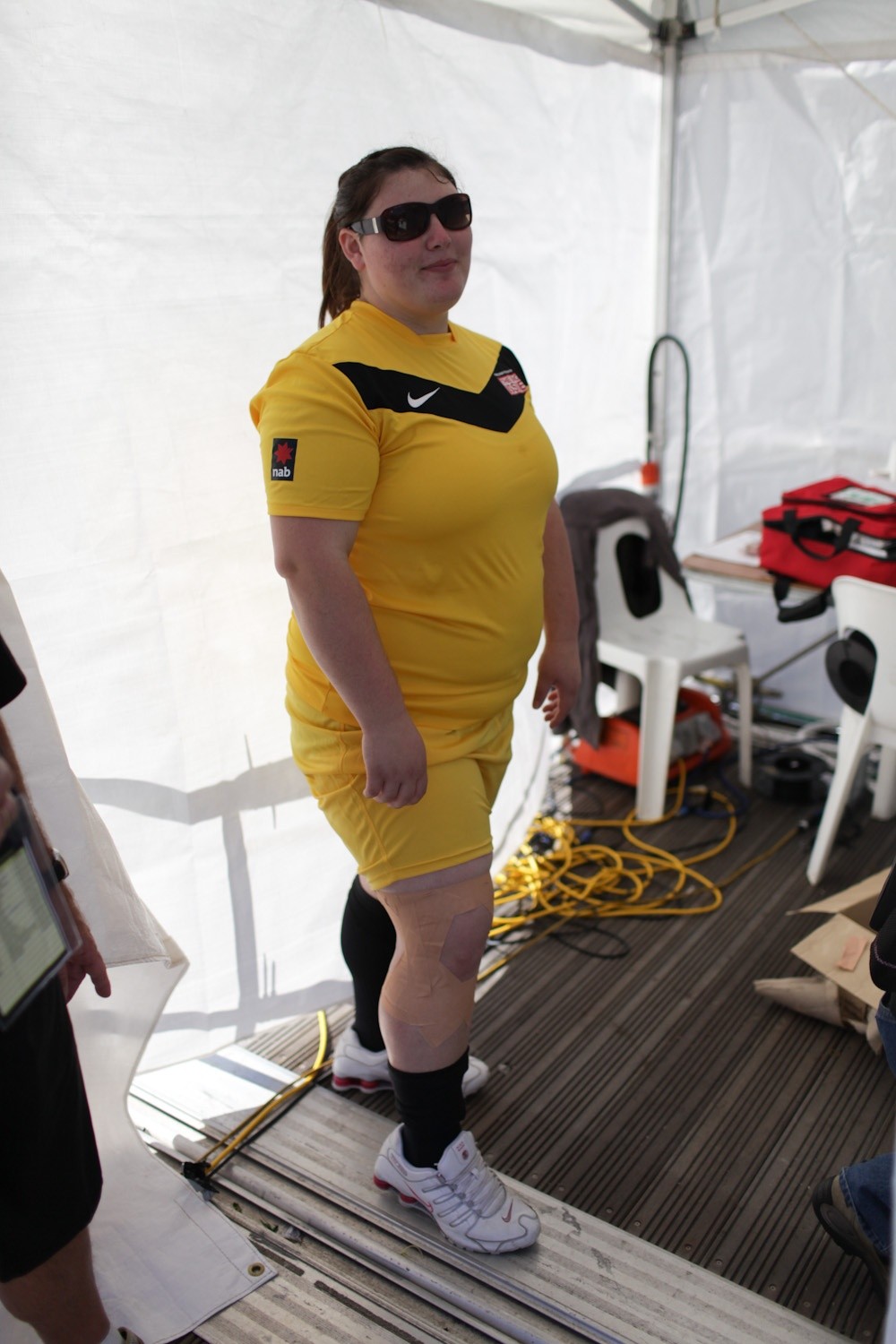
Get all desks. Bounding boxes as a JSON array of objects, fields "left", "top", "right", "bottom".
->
[{"left": 680, "top": 521, "right": 838, "bottom": 748}]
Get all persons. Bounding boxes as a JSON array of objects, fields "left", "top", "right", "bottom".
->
[
  {"left": 810, "top": 850, "right": 895, "bottom": 1287},
  {"left": 0, "top": 642, "right": 210, "bottom": 1344},
  {"left": 248, "top": 145, "right": 583, "bottom": 1254}
]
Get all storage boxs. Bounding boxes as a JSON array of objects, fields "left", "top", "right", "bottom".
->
[{"left": 782, "top": 864, "right": 891, "bottom": 1019}]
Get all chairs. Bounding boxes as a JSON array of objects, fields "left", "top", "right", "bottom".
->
[
  {"left": 558, "top": 491, "right": 754, "bottom": 821},
  {"left": 807, "top": 575, "right": 896, "bottom": 885}
]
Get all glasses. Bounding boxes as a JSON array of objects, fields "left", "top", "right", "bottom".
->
[{"left": 346, "top": 193, "right": 473, "bottom": 242}]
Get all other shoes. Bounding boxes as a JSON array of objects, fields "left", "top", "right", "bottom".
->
[{"left": 811, "top": 1172, "right": 890, "bottom": 1295}]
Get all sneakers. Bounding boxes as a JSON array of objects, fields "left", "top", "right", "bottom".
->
[
  {"left": 374, "top": 1120, "right": 541, "bottom": 1253},
  {"left": 329, "top": 1016, "right": 488, "bottom": 1099}
]
